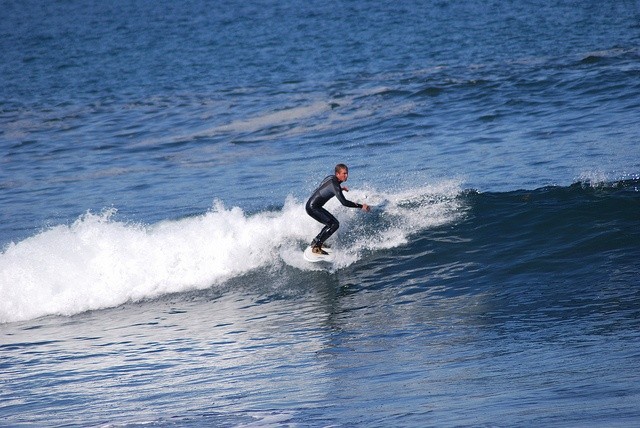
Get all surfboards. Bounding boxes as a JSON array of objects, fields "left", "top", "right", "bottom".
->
[{"left": 303, "top": 245, "right": 335, "bottom": 263}]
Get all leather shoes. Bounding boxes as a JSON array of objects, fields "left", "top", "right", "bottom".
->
[
  {"left": 311, "top": 239, "right": 330, "bottom": 248},
  {"left": 312, "top": 246, "right": 328, "bottom": 255}
]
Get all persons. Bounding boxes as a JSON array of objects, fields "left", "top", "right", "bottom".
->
[{"left": 305, "top": 163, "right": 369, "bottom": 255}]
